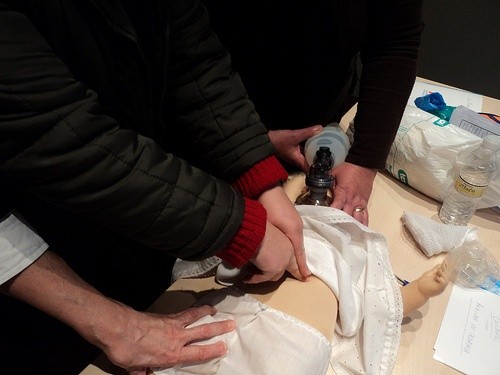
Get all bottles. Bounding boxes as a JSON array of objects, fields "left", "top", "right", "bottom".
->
[
  {"left": 305, "top": 119, "right": 350, "bottom": 170},
  {"left": 439, "top": 135, "right": 500, "bottom": 226}
]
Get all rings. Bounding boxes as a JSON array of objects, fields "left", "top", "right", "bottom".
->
[{"left": 353, "top": 207, "right": 365, "bottom": 215}]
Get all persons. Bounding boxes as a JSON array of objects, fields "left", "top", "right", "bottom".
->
[{"left": 0, "top": 0, "right": 451, "bottom": 375}]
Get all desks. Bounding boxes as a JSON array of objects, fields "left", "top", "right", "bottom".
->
[{"left": 78, "top": 74, "right": 500, "bottom": 375}]
